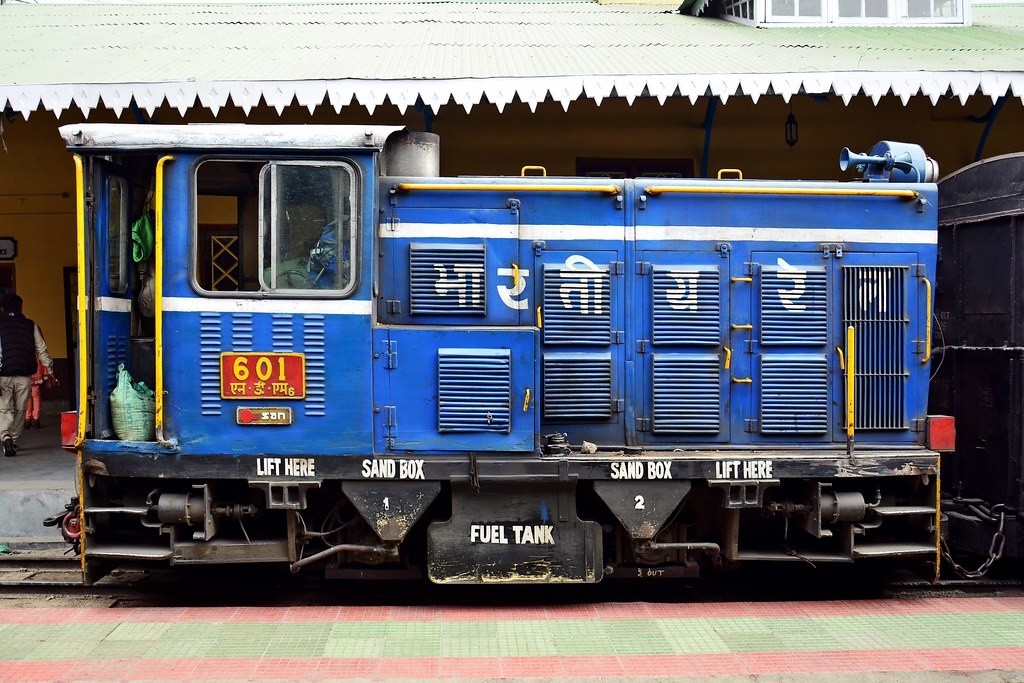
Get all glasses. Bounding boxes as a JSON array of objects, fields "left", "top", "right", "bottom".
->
[{"left": 286, "top": 210, "right": 310, "bottom": 226}]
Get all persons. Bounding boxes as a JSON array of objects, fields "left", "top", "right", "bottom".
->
[
  {"left": 0, "top": 293, "right": 60, "bottom": 457},
  {"left": 25, "top": 353, "right": 51, "bottom": 430}
]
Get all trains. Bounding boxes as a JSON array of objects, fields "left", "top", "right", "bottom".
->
[{"left": 41, "top": 123, "right": 1024, "bottom": 599}]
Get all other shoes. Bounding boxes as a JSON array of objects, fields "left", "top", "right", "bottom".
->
[
  {"left": 2, "top": 436, "right": 16, "bottom": 456},
  {"left": 11, "top": 445, "right": 19, "bottom": 451},
  {"left": 24, "top": 421, "right": 31, "bottom": 429},
  {"left": 31, "top": 418, "right": 40, "bottom": 429}
]
